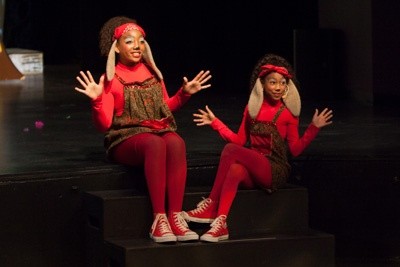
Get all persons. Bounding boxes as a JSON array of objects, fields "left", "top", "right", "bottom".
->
[
  {"left": 74, "top": 17, "right": 212, "bottom": 243},
  {"left": 182, "top": 55, "right": 333, "bottom": 243}
]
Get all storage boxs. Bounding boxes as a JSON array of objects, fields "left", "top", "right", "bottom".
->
[{"left": 7, "top": 48, "right": 43, "bottom": 74}]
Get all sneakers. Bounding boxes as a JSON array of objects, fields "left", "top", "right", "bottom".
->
[
  {"left": 182, "top": 197, "right": 217, "bottom": 223},
  {"left": 150, "top": 211, "right": 199, "bottom": 242},
  {"left": 200, "top": 215, "right": 229, "bottom": 241}
]
